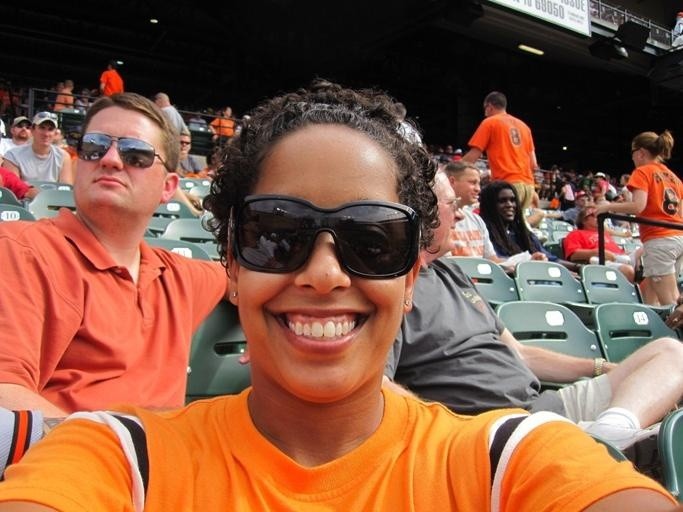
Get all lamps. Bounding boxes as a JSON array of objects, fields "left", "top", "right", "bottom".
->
[{"left": 611, "top": 21, "right": 650, "bottom": 50}]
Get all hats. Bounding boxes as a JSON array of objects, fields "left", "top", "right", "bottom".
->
[
  {"left": 11, "top": 117, "right": 31, "bottom": 128},
  {"left": 593, "top": 170, "right": 607, "bottom": 180},
  {"left": 30, "top": 111, "right": 59, "bottom": 128}
]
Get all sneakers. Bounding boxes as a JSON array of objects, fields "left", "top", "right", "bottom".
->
[{"left": 588, "top": 423, "right": 664, "bottom": 472}]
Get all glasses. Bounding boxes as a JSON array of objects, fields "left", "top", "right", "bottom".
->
[
  {"left": 630, "top": 148, "right": 639, "bottom": 156},
  {"left": 437, "top": 194, "right": 464, "bottom": 210},
  {"left": 77, "top": 130, "right": 172, "bottom": 170},
  {"left": 177, "top": 138, "right": 194, "bottom": 147},
  {"left": 237, "top": 193, "right": 422, "bottom": 276}
]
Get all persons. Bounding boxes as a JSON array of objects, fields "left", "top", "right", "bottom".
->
[
  {"left": 1, "top": 60, "right": 253, "bottom": 218},
  {"left": 382, "top": 164, "right": 683, "bottom": 490},
  {"left": 383, "top": 92, "right": 683, "bottom": 451},
  {"left": 0, "top": 405, "right": 51, "bottom": 481},
  {"left": 0, "top": 91, "right": 254, "bottom": 418},
  {"left": 0, "top": 78, "right": 683, "bottom": 512}
]
